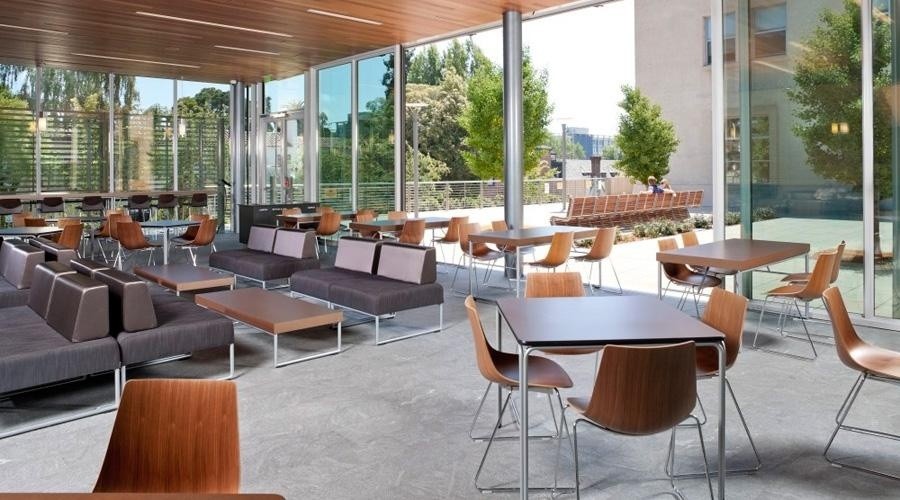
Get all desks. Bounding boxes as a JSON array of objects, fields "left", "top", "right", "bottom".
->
[
  {"left": 0, "top": 492, "right": 284, "bottom": 500},
  {"left": 656, "top": 237, "right": 811, "bottom": 353},
  {"left": 494, "top": 297, "right": 727, "bottom": 500}
]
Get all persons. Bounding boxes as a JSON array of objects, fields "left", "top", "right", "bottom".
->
[
  {"left": 660, "top": 179, "right": 671, "bottom": 191},
  {"left": 638, "top": 176, "right": 676, "bottom": 197}
]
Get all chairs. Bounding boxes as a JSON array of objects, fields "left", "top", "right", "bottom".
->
[
  {"left": 753, "top": 250, "right": 839, "bottom": 356},
  {"left": 658, "top": 239, "right": 724, "bottom": 320},
  {"left": 681, "top": 231, "right": 739, "bottom": 295},
  {"left": 90, "top": 377, "right": 240, "bottom": 493},
  {"left": 465, "top": 295, "right": 578, "bottom": 493},
  {"left": 821, "top": 287, "right": 900, "bottom": 484},
  {"left": 776, "top": 240, "right": 846, "bottom": 331},
  {"left": 663, "top": 287, "right": 763, "bottom": 480},
  {"left": 549, "top": 340, "right": 716, "bottom": 499}
]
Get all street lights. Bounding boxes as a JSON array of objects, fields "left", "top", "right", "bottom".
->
[
  {"left": 404, "top": 102, "right": 431, "bottom": 219},
  {"left": 561, "top": 122, "right": 570, "bottom": 211},
  {"left": 828, "top": 115, "right": 850, "bottom": 177}
]
